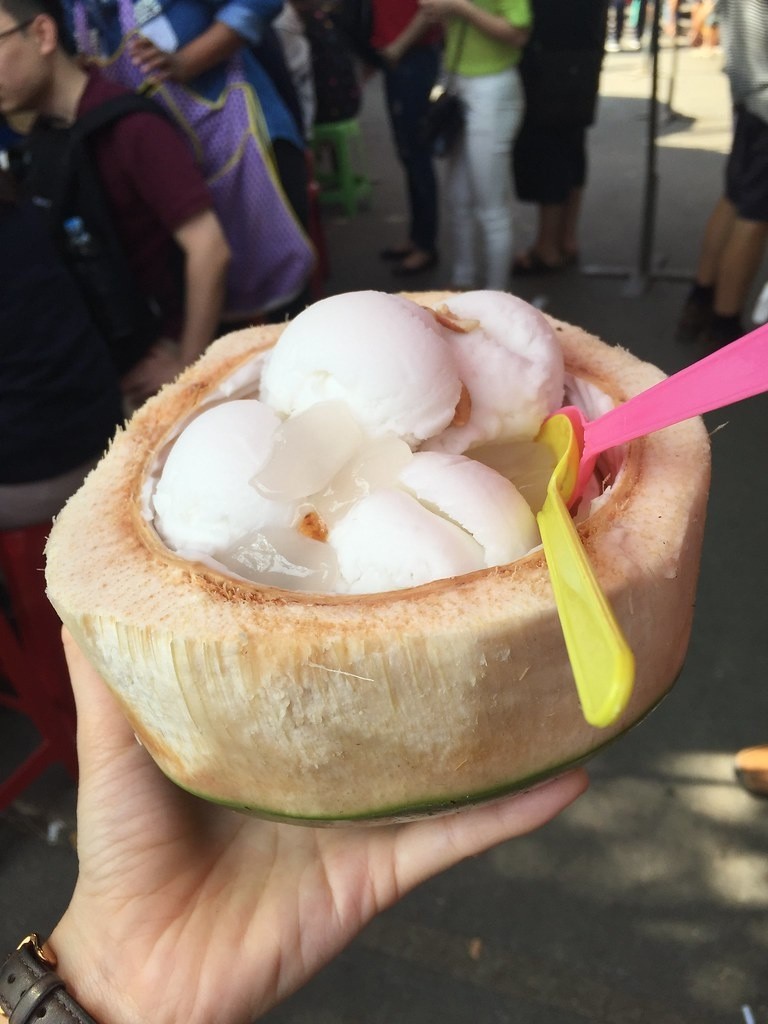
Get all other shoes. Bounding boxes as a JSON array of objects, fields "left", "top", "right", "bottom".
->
[
  {"left": 391, "top": 254, "right": 438, "bottom": 278},
  {"left": 676, "top": 285, "right": 749, "bottom": 360},
  {"left": 510, "top": 244, "right": 554, "bottom": 278},
  {"left": 379, "top": 243, "right": 414, "bottom": 261},
  {"left": 550, "top": 242, "right": 581, "bottom": 272}
]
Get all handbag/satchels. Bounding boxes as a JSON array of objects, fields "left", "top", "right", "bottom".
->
[{"left": 405, "top": 89, "right": 466, "bottom": 155}]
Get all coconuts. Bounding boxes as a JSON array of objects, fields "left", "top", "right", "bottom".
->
[{"left": 42, "top": 290, "right": 712, "bottom": 834}]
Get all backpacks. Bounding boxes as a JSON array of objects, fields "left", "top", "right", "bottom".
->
[{"left": 1, "top": 93, "right": 204, "bottom": 487}]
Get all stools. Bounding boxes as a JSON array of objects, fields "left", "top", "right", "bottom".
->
[{"left": 307, "top": 120, "right": 376, "bottom": 218}]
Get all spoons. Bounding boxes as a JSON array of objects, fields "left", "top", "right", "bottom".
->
[
  {"left": 535, "top": 414, "right": 636, "bottom": 729},
  {"left": 543, "top": 323, "right": 768, "bottom": 513}
]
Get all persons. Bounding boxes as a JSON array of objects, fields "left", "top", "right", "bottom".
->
[
  {"left": 0, "top": 0, "right": 768, "bottom": 531},
  {"left": 0, "top": 623, "right": 592, "bottom": 1024}
]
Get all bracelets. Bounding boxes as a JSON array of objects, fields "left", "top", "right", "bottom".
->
[{"left": 0, "top": 931, "right": 98, "bottom": 1024}]
